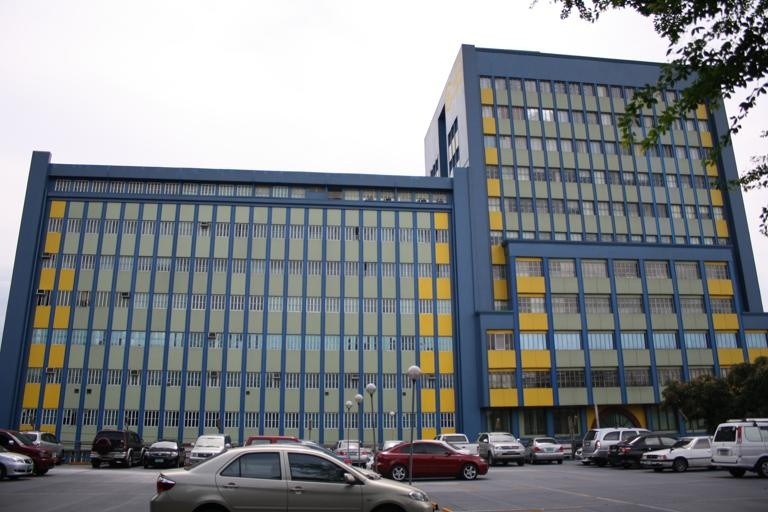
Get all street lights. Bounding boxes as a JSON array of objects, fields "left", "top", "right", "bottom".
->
[
  {"left": 389, "top": 411, "right": 396, "bottom": 442},
  {"left": 355, "top": 393, "right": 362, "bottom": 467},
  {"left": 346, "top": 400, "right": 351, "bottom": 459},
  {"left": 365, "top": 382, "right": 377, "bottom": 474},
  {"left": 406, "top": 366, "right": 421, "bottom": 485}
]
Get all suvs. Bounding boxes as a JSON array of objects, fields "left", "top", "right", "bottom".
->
[
  {"left": 0, "top": 428, "right": 54, "bottom": 477},
  {"left": 709, "top": 417, "right": 768, "bottom": 479},
  {"left": 583, "top": 427, "right": 654, "bottom": 467}
]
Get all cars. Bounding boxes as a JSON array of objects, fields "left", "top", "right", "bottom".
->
[
  {"left": 0, "top": 446, "right": 34, "bottom": 480},
  {"left": 148, "top": 443, "right": 440, "bottom": 511},
  {"left": 17, "top": 430, "right": 66, "bottom": 466},
  {"left": 606, "top": 432, "right": 679, "bottom": 472},
  {"left": 639, "top": 434, "right": 729, "bottom": 471},
  {"left": 575, "top": 447, "right": 593, "bottom": 465}
]
